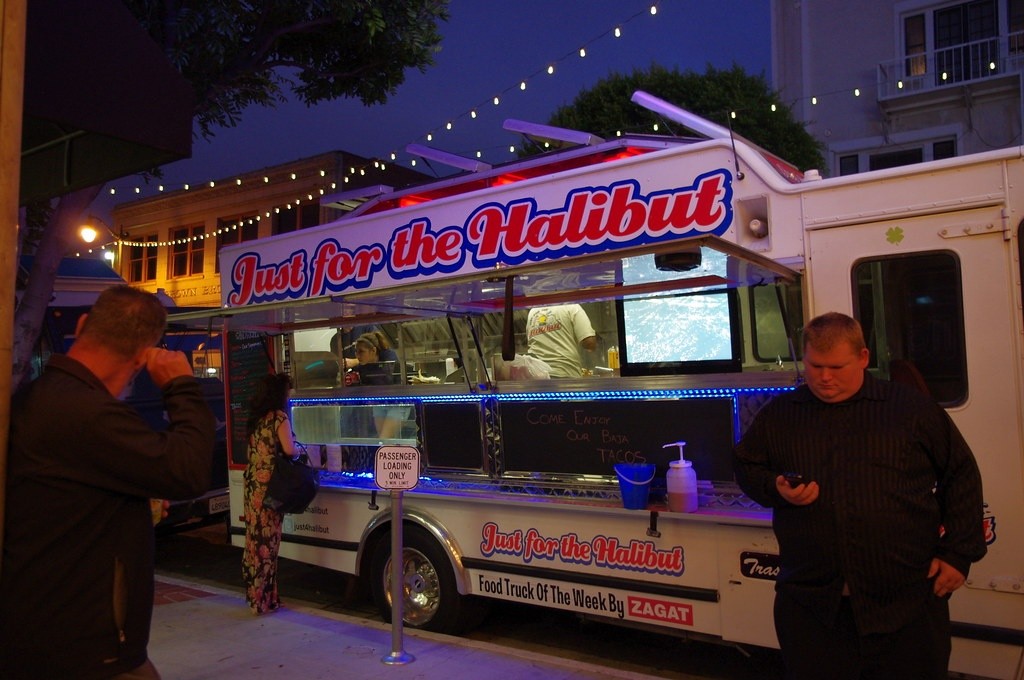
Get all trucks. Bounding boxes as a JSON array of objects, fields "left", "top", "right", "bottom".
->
[{"left": 214, "top": 82, "right": 1024, "bottom": 680}]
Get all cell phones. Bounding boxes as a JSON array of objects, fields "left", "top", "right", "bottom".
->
[{"left": 784, "top": 472, "right": 804, "bottom": 489}]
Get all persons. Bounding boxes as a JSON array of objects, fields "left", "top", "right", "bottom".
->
[
  {"left": 239, "top": 372, "right": 303, "bottom": 615},
  {"left": 331, "top": 294, "right": 425, "bottom": 479},
  {"left": 734, "top": 311, "right": 988, "bottom": 678},
  {"left": 523, "top": 306, "right": 608, "bottom": 381},
  {"left": 2, "top": 287, "right": 219, "bottom": 678}
]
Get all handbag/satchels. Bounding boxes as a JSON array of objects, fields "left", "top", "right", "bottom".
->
[{"left": 268, "top": 441, "right": 320, "bottom": 508}]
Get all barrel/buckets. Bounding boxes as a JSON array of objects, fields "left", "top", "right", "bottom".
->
[{"left": 614, "top": 463, "right": 656, "bottom": 510}]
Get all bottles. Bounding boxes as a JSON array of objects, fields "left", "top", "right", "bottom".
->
[{"left": 608, "top": 345, "right": 619, "bottom": 368}]
